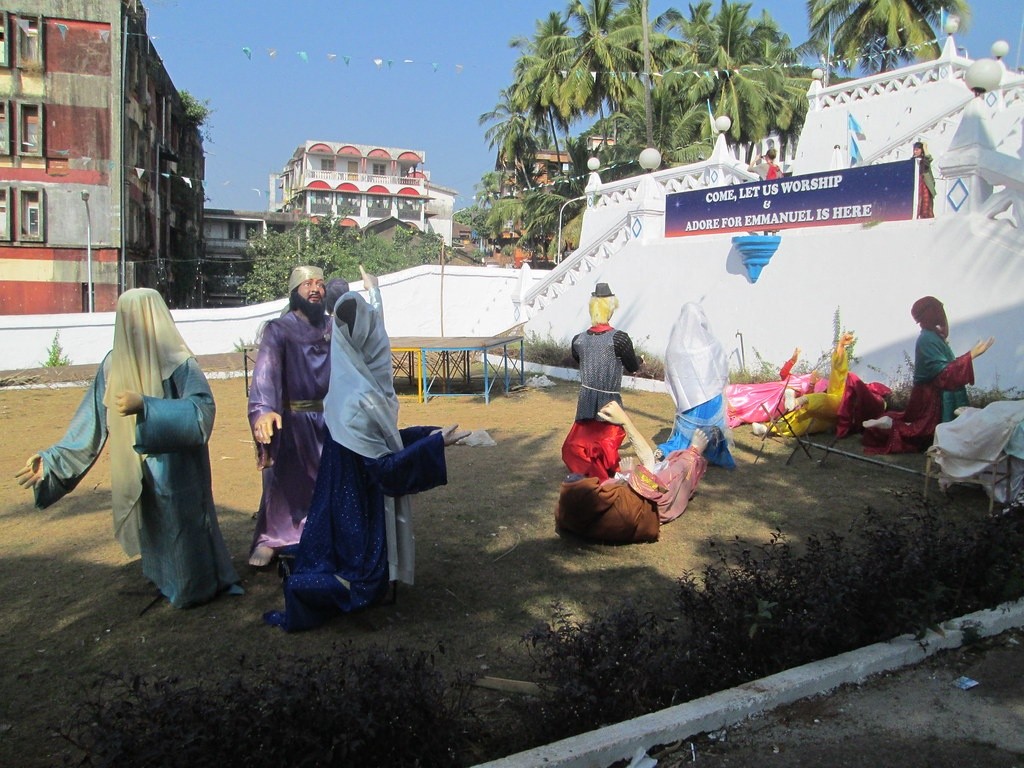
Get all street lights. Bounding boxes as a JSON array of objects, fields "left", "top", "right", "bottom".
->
[
  {"left": 450, "top": 208, "right": 466, "bottom": 247},
  {"left": 556, "top": 195, "right": 586, "bottom": 266},
  {"left": 81, "top": 189, "right": 95, "bottom": 313}
]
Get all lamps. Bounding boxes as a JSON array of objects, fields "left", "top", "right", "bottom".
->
[
  {"left": 715, "top": 116, "right": 731, "bottom": 134},
  {"left": 992, "top": 40, "right": 1009, "bottom": 60},
  {"left": 966, "top": 57, "right": 1001, "bottom": 97},
  {"left": 639, "top": 148, "right": 662, "bottom": 173},
  {"left": 587, "top": 158, "right": 600, "bottom": 172},
  {"left": 944, "top": 22, "right": 958, "bottom": 36},
  {"left": 811, "top": 69, "right": 823, "bottom": 80}
]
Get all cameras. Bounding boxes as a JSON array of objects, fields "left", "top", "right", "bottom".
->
[{"left": 761, "top": 156, "right": 763, "bottom": 158}]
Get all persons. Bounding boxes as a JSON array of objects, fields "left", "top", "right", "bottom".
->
[
  {"left": 752, "top": 330, "right": 891, "bottom": 439},
  {"left": 15, "top": 288, "right": 246, "bottom": 609},
  {"left": 726, "top": 331, "right": 828, "bottom": 428},
  {"left": 746, "top": 149, "right": 779, "bottom": 181},
  {"left": 326, "top": 265, "right": 384, "bottom": 318},
  {"left": 556, "top": 401, "right": 709, "bottom": 543},
  {"left": 657, "top": 304, "right": 737, "bottom": 470},
  {"left": 912, "top": 142, "right": 937, "bottom": 219},
  {"left": 246, "top": 265, "right": 332, "bottom": 566},
  {"left": 561, "top": 284, "right": 645, "bottom": 482},
  {"left": 263, "top": 291, "right": 471, "bottom": 634},
  {"left": 862, "top": 295, "right": 995, "bottom": 455}
]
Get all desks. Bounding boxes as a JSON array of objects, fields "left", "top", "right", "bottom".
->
[{"left": 243, "top": 336, "right": 525, "bottom": 406}]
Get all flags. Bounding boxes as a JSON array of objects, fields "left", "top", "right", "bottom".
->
[
  {"left": 708, "top": 102, "right": 718, "bottom": 133},
  {"left": 848, "top": 113, "right": 866, "bottom": 168}
]
